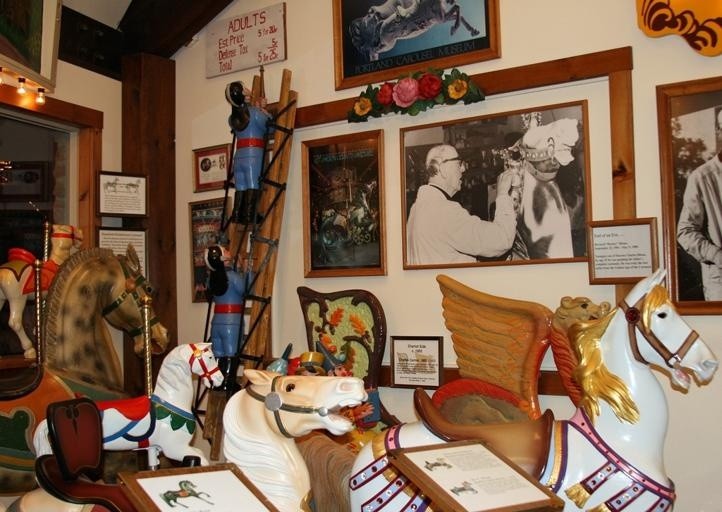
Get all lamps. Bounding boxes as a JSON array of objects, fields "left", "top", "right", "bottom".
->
[
  {"left": 35, "top": 86, "right": 46, "bottom": 106},
  {"left": 16, "top": 77, "right": 27, "bottom": 97}
]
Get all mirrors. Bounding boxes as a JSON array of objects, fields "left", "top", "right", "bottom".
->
[{"left": 1, "top": 83, "right": 104, "bottom": 269}]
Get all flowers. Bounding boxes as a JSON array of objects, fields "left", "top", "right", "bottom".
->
[{"left": 347, "top": 67, "right": 485, "bottom": 123}]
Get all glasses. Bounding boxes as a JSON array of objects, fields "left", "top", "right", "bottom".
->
[{"left": 439, "top": 155, "right": 464, "bottom": 166}]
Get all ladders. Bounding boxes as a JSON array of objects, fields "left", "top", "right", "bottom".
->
[{"left": 201, "top": 69, "right": 298, "bottom": 461}]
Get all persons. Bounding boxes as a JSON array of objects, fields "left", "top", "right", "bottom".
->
[
  {"left": 676, "top": 108, "right": 722, "bottom": 301},
  {"left": 223, "top": 81, "right": 274, "bottom": 226},
  {"left": 407, "top": 145, "right": 519, "bottom": 266},
  {"left": 203, "top": 244, "right": 252, "bottom": 392},
  {"left": 500, "top": 120, "right": 579, "bottom": 262}
]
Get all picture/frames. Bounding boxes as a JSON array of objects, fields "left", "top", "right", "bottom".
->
[
  {"left": 399, "top": 98, "right": 591, "bottom": 271},
  {"left": 654, "top": 77, "right": 721, "bottom": 315},
  {"left": 388, "top": 437, "right": 565, "bottom": 511},
  {"left": 1, "top": 160, "right": 50, "bottom": 266},
  {"left": 331, "top": 0, "right": 501, "bottom": 91},
  {"left": 585, "top": 216, "right": 660, "bottom": 285},
  {"left": 94, "top": 170, "right": 149, "bottom": 218},
  {"left": 390, "top": 335, "right": 443, "bottom": 390},
  {"left": 192, "top": 143, "right": 232, "bottom": 193},
  {"left": 301, "top": 129, "right": 390, "bottom": 277},
  {"left": 97, "top": 226, "right": 151, "bottom": 288},
  {"left": 187, "top": 196, "right": 233, "bottom": 303},
  {"left": 114, "top": 463, "right": 280, "bottom": 512}
]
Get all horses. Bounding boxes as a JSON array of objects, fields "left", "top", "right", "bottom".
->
[
  {"left": 160, "top": 480, "right": 215, "bottom": 510},
  {"left": 369, "top": 0, "right": 479, "bottom": 62}
]
